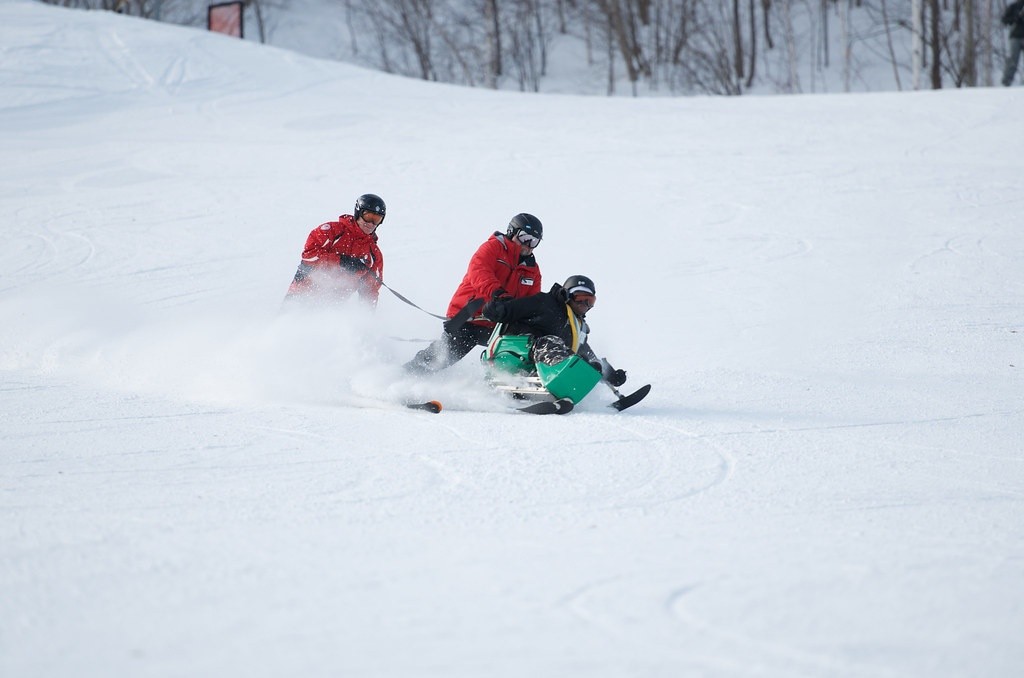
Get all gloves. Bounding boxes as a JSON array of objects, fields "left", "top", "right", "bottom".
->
[
  {"left": 482, "top": 301, "right": 507, "bottom": 323},
  {"left": 339, "top": 254, "right": 370, "bottom": 275},
  {"left": 607, "top": 369, "right": 627, "bottom": 387},
  {"left": 492, "top": 289, "right": 513, "bottom": 302}
]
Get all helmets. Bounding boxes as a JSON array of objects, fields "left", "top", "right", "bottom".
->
[
  {"left": 354, "top": 194, "right": 386, "bottom": 217},
  {"left": 506, "top": 212, "right": 543, "bottom": 240},
  {"left": 563, "top": 275, "right": 595, "bottom": 295}
]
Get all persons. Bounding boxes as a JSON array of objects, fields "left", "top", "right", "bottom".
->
[
  {"left": 1000, "top": 0, "right": 1024, "bottom": 87},
  {"left": 287, "top": 194, "right": 387, "bottom": 312},
  {"left": 401, "top": 214, "right": 626, "bottom": 411}
]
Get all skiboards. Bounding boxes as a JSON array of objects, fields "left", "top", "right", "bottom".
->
[
  {"left": 402, "top": 387, "right": 576, "bottom": 420},
  {"left": 557, "top": 383, "right": 659, "bottom": 424}
]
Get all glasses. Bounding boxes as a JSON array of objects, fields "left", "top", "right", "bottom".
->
[
  {"left": 569, "top": 294, "right": 596, "bottom": 307},
  {"left": 358, "top": 208, "right": 385, "bottom": 225},
  {"left": 512, "top": 228, "right": 541, "bottom": 248}
]
